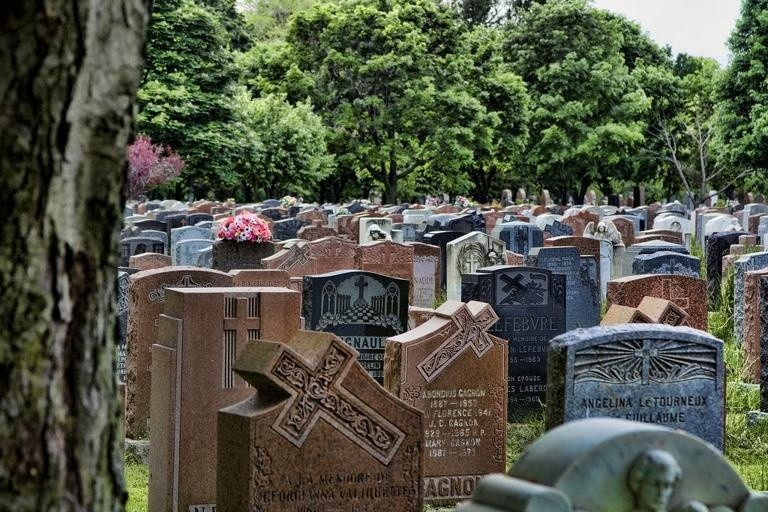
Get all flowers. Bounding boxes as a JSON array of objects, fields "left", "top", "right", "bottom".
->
[{"left": 217, "top": 213, "right": 272, "bottom": 243}]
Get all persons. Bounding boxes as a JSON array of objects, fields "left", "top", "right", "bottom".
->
[
  {"left": 627, "top": 449, "right": 683, "bottom": 510},
  {"left": 366, "top": 224, "right": 393, "bottom": 242},
  {"left": 594, "top": 222, "right": 608, "bottom": 238},
  {"left": 488, "top": 251, "right": 503, "bottom": 266}
]
